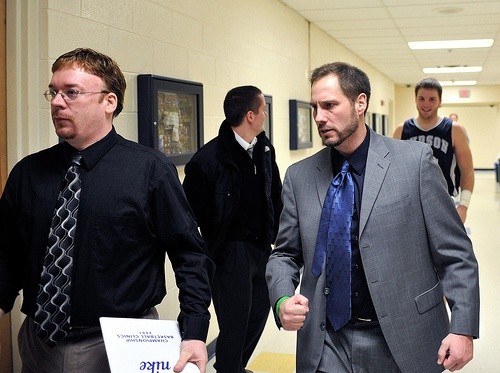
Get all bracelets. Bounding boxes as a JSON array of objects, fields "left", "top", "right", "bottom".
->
[
  {"left": 276, "top": 295, "right": 291, "bottom": 316},
  {"left": 459, "top": 189, "right": 472, "bottom": 209}
]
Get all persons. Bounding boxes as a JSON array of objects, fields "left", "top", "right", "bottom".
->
[
  {"left": 182, "top": 85, "right": 283, "bottom": 373},
  {"left": 266, "top": 61, "right": 480, "bottom": 373},
  {"left": 0, "top": 48, "right": 212, "bottom": 373},
  {"left": 449, "top": 113, "right": 469, "bottom": 193},
  {"left": 393, "top": 77, "right": 474, "bottom": 224}
]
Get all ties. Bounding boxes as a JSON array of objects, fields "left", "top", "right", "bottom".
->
[
  {"left": 32, "top": 154, "right": 82, "bottom": 350},
  {"left": 312, "top": 160, "right": 355, "bottom": 331}
]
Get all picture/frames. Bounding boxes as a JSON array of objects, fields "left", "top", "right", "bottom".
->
[
  {"left": 364, "top": 111, "right": 370, "bottom": 127},
  {"left": 262, "top": 95, "right": 274, "bottom": 145},
  {"left": 137, "top": 73, "right": 204, "bottom": 167},
  {"left": 288, "top": 99, "right": 313, "bottom": 151},
  {"left": 372, "top": 113, "right": 380, "bottom": 135},
  {"left": 381, "top": 114, "right": 389, "bottom": 136}
]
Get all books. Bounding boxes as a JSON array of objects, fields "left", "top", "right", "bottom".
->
[{"left": 100, "top": 317, "right": 201, "bottom": 373}]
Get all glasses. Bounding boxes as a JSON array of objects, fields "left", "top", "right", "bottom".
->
[{"left": 44, "top": 88, "right": 108, "bottom": 101}]
